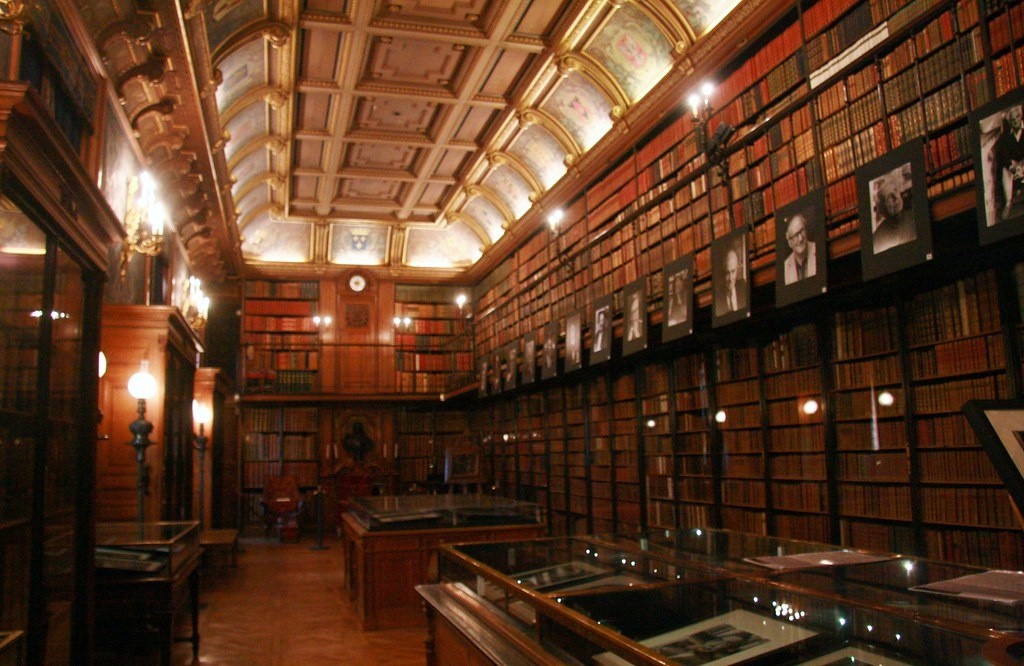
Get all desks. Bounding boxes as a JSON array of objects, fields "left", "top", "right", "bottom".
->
[
  {"left": 44, "top": 522, "right": 201, "bottom": 666},
  {"left": 340, "top": 514, "right": 548, "bottom": 633},
  {"left": 413, "top": 535, "right": 1024, "bottom": 666},
  {"left": 195, "top": 528, "right": 239, "bottom": 570}
]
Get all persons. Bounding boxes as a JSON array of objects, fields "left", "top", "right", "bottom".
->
[
  {"left": 714, "top": 249, "right": 747, "bottom": 315},
  {"left": 873, "top": 183, "right": 915, "bottom": 253},
  {"left": 594, "top": 311, "right": 608, "bottom": 352},
  {"left": 627, "top": 295, "right": 642, "bottom": 342},
  {"left": 669, "top": 276, "right": 686, "bottom": 327},
  {"left": 784, "top": 214, "right": 816, "bottom": 286},
  {"left": 655, "top": 634, "right": 746, "bottom": 666},
  {"left": 991, "top": 107, "right": 1024, "bottom": 220}
]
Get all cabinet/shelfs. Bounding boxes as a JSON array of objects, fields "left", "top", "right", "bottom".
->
[
  {"left": 94, "top": 305, "right": 234, "bottom": 617},
  {"left": 0, "top": 81, "right": 128, "bottom": 666},
  {"left": 240, "top": 0, "right": 1024, "bottom": 571}
]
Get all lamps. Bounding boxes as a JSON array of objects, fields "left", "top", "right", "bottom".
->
[
  {"left": 127, "top": 358, "right": 158, "bottom": 522},
  {"left": 455, "top": 293, "right": 475, "bottom": 340},
  {"left": 545, "top": 204, "right": 575, "bottom": 273},
  {"left": 685, "top": 82, "right": 736, "bottom": 187},
  {"left": 192, "top": 403, "right": 213, "bottom": 530}
]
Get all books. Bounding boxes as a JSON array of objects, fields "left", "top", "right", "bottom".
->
[{"left": 0, "top": 1, "right": 1024, "bottom": 576}]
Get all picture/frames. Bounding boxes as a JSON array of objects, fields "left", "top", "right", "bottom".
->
[
  {"left": 662, "top": 252, "right": 693, "bottom": 343},
  {"left": 621, "top": 273, "right": 648, "bottom": 357},
  {"left": 711, "top": 223, "right": 753, "bottom": 328},
  {"left": 541, "top": 319, "right": 558, "bottom": 379},
  {"left": 478, "top": 330, "right": 536, "bottom": 399},
  {"left": 469, "top": 557, "right": 648, "bottom": 627},
  {"left": 585, "top": 601, "right": 925, "bottom": 666},
  {"left": 775, "top": 186, "right": 828, "bottom": 308},
  {"left": 969, "top": 85, "right": 1024, "bottom": 249},
  {"left": 589, "top": 293, "right": 612, "bottom": 366},
  {"left": 565, "top": 306, "right": 582, "bottom": 373},
  {"left": 960, "top": 399, "right": 1024, "bottom": 518},
  {"left": 855, "top": 135, "right": 934, "bottom": 283}
]
